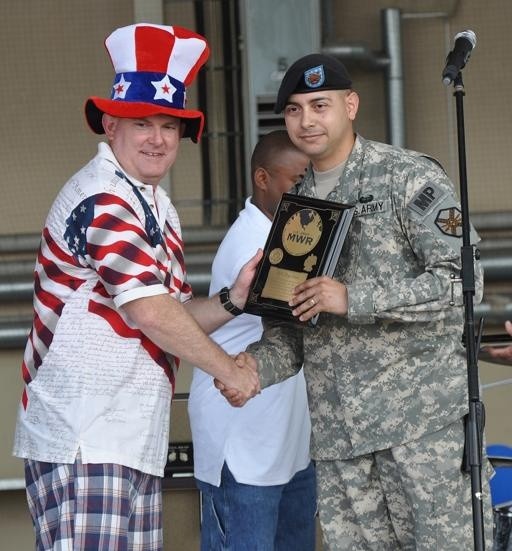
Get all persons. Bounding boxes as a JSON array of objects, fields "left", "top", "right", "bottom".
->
[
  {"left": 484, "top": 319, "right": 512, "bottom": 363},
  {"left": 217, "top": 54, "right": 495, "bottom": 551},
  {"left": 186, "top": 130, "right": 317, "bottom": 550},
  {"left": 7, "top": 95, "right": 264, "bottom": 550}
]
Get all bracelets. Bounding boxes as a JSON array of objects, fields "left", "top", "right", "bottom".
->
[{"left": 220, "top": 287, "right": 243, "bottom": 316}]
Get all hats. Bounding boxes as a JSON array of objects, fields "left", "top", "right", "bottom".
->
[
  {"left": 84, "top": 22, "right": 209, "bottom": 144},
  {"left": 274, "top": 53, "right": 352, "bottom": 115}
]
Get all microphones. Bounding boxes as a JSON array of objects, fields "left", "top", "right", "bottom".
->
[{"left": 442, "top": 29, "right": 476, "bottom": 86}]
